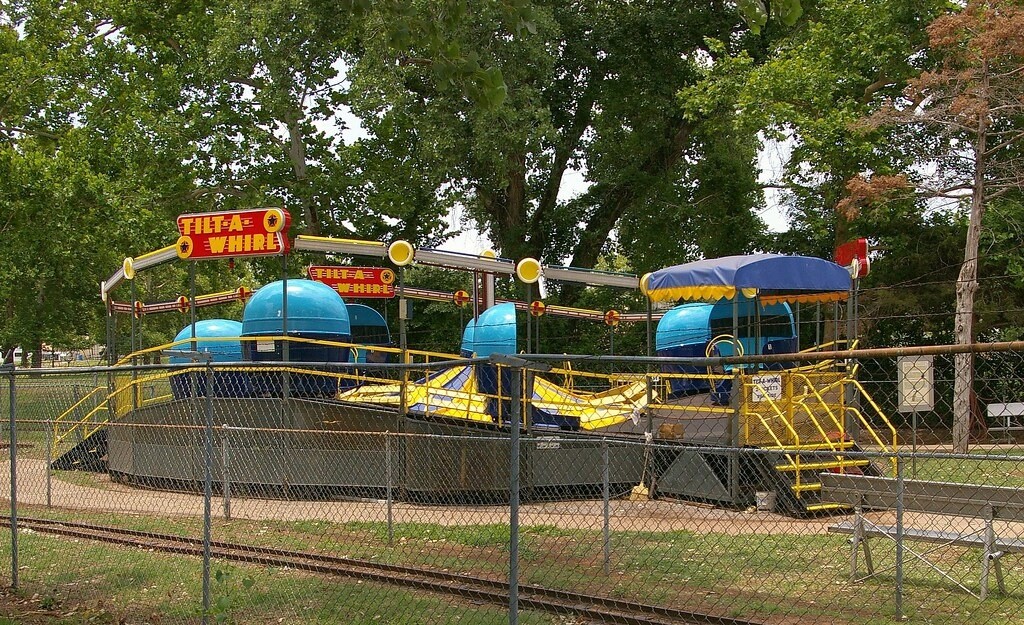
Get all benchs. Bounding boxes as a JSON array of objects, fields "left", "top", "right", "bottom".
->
[
  {"left": 986, "top": 402, "right": 1024, "bottom": 445},
  {"left": 819, "top": 472, "right": 1024, "bottom": 602}
]
